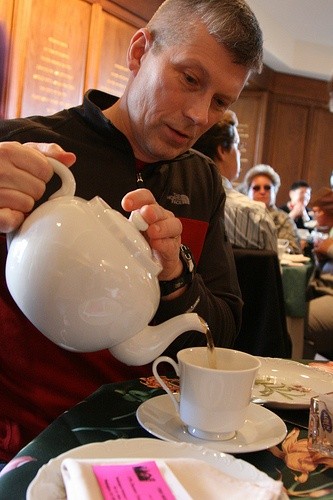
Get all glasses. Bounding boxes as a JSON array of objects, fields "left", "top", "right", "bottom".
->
[{"left": 250, "top": 186, "right": 275, "bottom": 190}]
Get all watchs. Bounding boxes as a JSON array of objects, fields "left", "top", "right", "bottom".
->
[{"left": 154, "top": 243, "right": 195, "bottom": 299}]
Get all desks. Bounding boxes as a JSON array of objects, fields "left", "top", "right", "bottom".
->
[{"left": 0, "top": 248, "right": 333, "bottom": 500}]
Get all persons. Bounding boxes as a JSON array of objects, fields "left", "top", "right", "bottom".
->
[
  {"left": 238, "top": 164, "right": 304, "bottom": 255},
  {"left": 275, "top": 181, "right": 316, "bottom": 233},
  {"left": 0, "top": 0, "right": 267, "bottom": 478},
  {"left": 191, "top": 105, "right": 293, "bottom": 359},
  {"left": 287, "top": 189, "right": 333, "bottom": 362}
]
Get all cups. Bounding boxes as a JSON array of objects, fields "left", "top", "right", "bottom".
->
[{"left": 152, "top": 347, "right": 261, "bottom": 441}]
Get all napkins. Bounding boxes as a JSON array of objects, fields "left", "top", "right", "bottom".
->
[{"left": 59, "top": 457, "right": 274, "bottom": 500}]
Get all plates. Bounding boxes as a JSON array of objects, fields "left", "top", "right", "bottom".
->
[
  {"left": 249, "top": 355, "right": 333, "bottom": 410},
  {"left": 26, "top": 438, "right": 291, "bottom": 499},
  {"left": 135, "top": 392, "right": 288, "bottom": 453}
]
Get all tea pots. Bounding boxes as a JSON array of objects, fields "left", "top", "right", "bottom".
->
[{"left": 5, "top": 155, "right": 208, "bottom": 367}]
